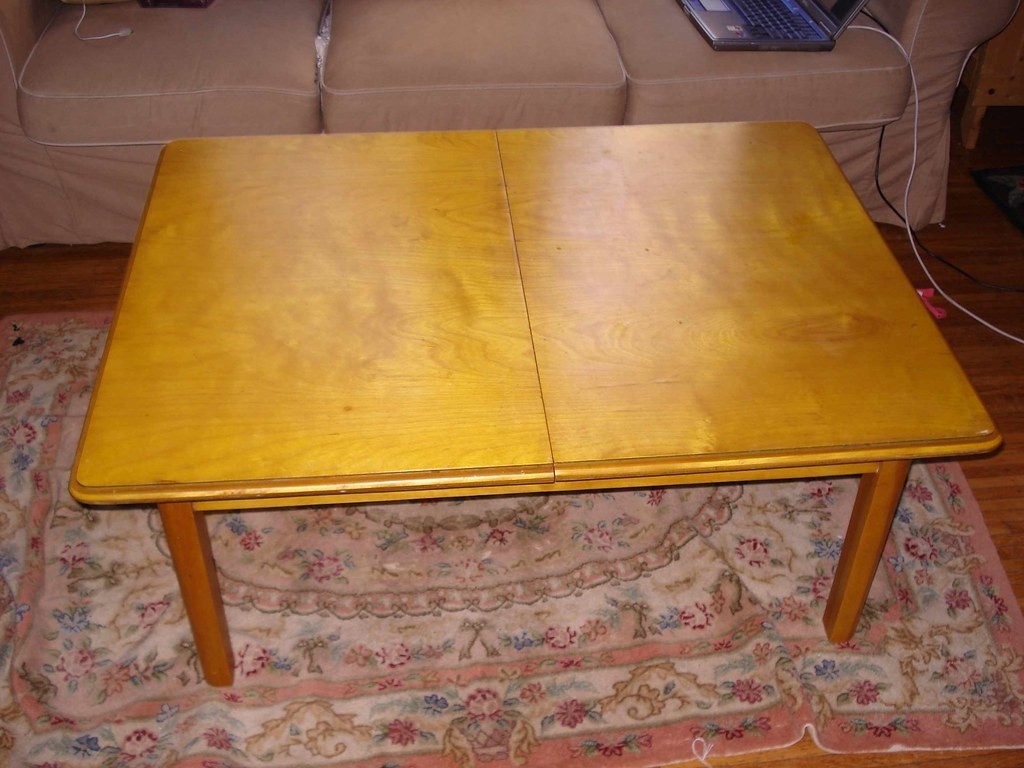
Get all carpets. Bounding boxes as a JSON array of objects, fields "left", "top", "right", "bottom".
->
[{"left": 2, "top": 312, "right": 1024, "bottom": 767}]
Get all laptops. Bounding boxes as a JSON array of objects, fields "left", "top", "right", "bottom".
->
[{"left": 676, "top": 0, "right": 869, "bottom": 52}]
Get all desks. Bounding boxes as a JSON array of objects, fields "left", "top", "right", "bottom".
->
[{"left": 70, "top": 122, "right": 1004, "bottom": 688}]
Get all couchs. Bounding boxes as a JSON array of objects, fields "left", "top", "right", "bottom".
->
[{"left": 1, "top": 0, "right": 1020, "bottom": 255}]
